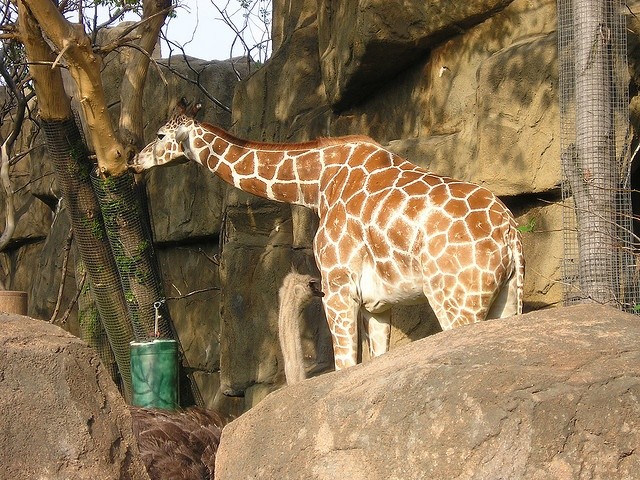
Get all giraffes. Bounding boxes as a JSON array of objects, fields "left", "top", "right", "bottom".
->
[{"left": 129, "top": 97, "right": 524, "bottom": 373}]
